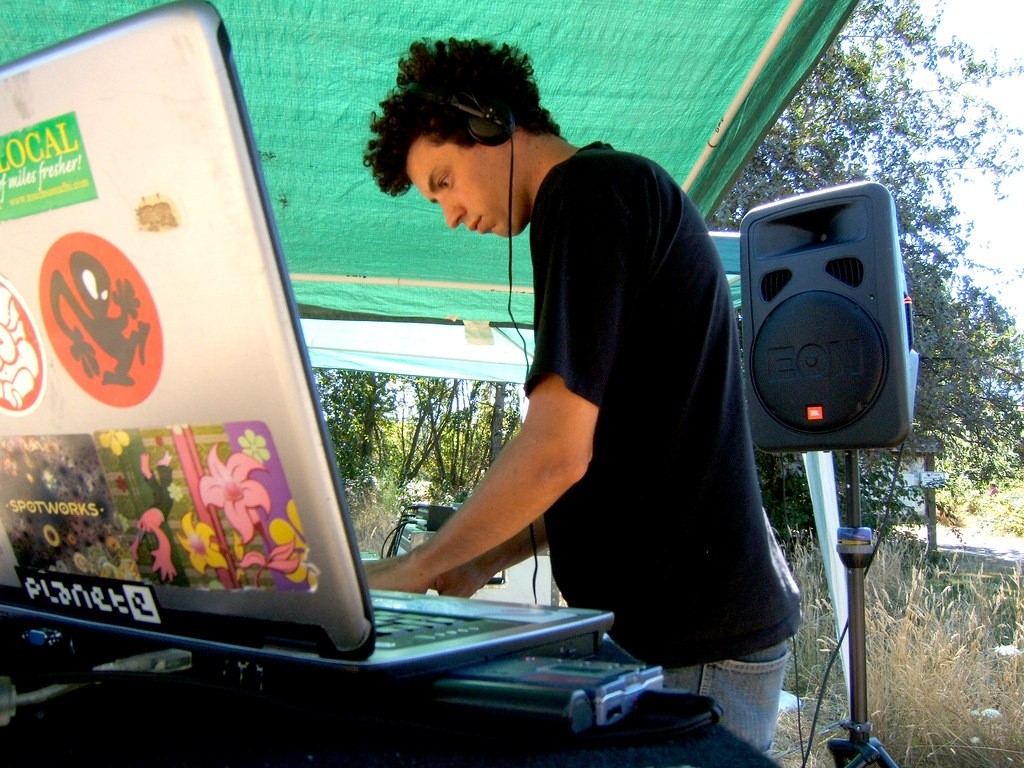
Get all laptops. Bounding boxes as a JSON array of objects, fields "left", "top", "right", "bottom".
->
[{"left": 0, "top": 1, "right": 618, "bottom": 683}]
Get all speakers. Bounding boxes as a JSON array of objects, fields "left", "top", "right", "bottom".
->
[{"left": 743, "top": 181, "right": 920, "bottom": 457}]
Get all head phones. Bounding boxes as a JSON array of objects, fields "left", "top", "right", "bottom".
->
[{"left": 436, "top": 47, "right": 516, "bottom": 146}]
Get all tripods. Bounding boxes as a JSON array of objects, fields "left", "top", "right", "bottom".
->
[{"left": 825, "top": 448, "right": 895, "bottom": 768}]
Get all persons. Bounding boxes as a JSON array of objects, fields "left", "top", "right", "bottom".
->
[
  {"left": 360, "top": 34, "right": 803, "bottom": 754},
  {"left": 989, "top": 482, "right": 998, "bottom": 495}
]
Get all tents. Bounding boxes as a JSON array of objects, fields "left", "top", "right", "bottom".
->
[{"left": 301, "top": 229, "right": 854, "bottom": 715}]
